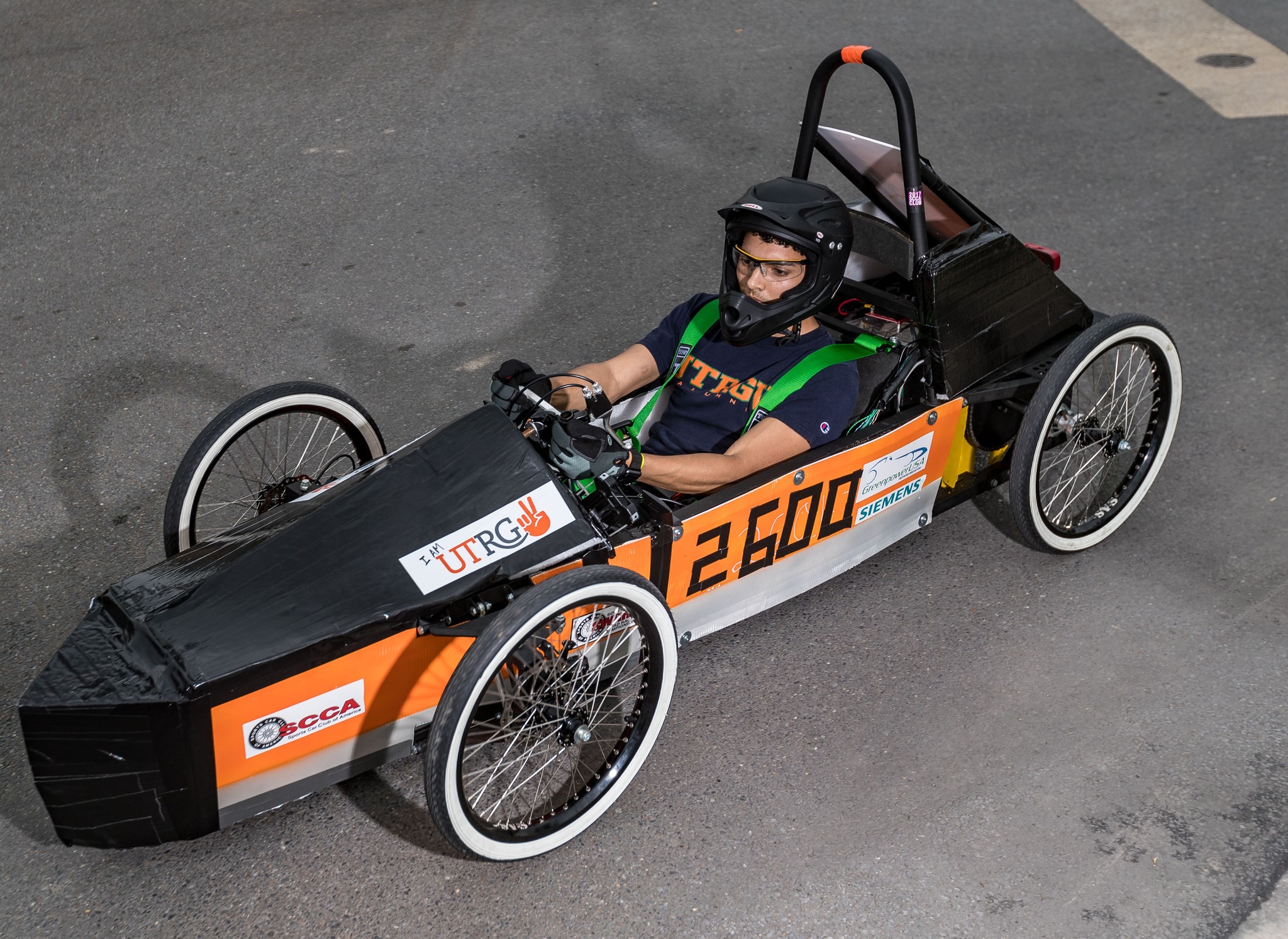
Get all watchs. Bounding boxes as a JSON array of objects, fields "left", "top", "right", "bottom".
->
[{"left": 617, "top": 449, "right": 642, "bottom": 485}]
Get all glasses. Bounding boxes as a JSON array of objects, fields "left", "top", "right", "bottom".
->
[{"left": 732, "top": 243, "right": 807, "bottom": 282}]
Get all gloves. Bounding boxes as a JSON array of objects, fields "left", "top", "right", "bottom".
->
[
  {"left": 491, "top": 358, "right": 552, "bottom": 421},
  {"left": 549, "top": 417, "right": 643, "bottom": 487}
]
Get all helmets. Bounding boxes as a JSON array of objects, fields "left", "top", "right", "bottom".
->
[{"left": 717, "top": 176, "right": 855, "bottom": 346}]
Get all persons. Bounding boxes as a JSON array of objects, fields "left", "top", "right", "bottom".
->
[{"left": 491, "top": 176, "right": 860, "bottom": 512}]
